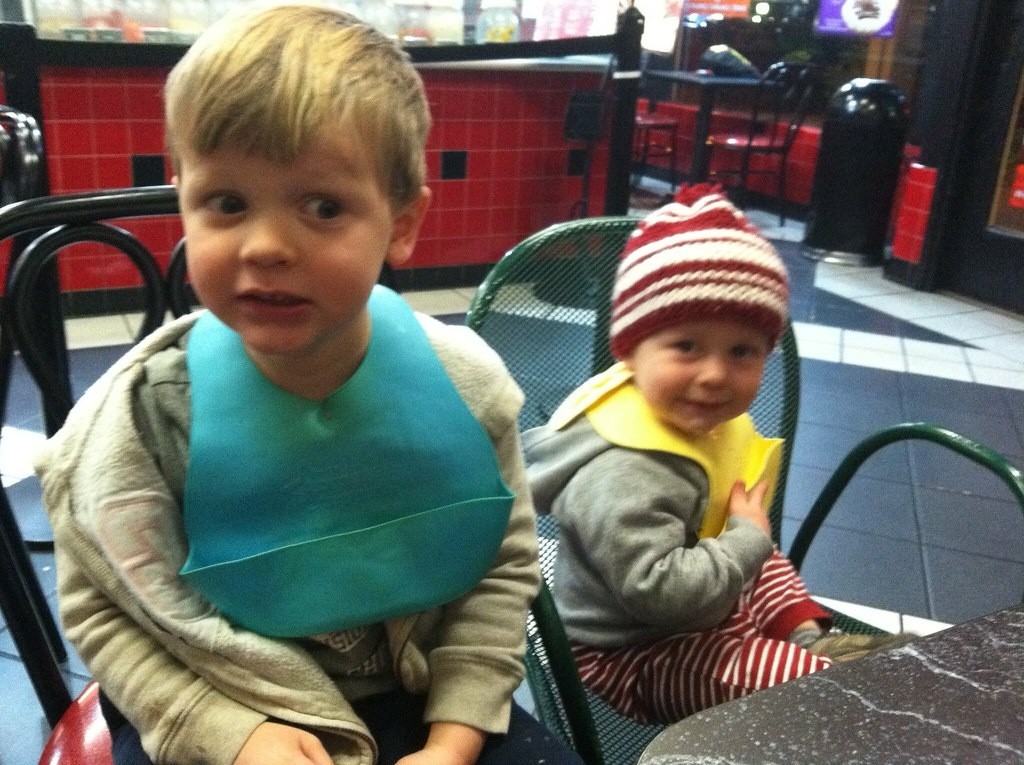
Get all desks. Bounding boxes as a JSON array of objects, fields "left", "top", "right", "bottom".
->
[
  {"left": 636, "top": 600, "right": 1024, "bottom": 765},
  {"left": 648, "top": 68, "right": 775, "bottom": 177}
]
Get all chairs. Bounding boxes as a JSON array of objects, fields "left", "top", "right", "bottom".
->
[
  {"left": 706, "top": 60, "right": 825, "bottom": 222},
  {"left": 0, "top": 184, "right": 1024, "bottom": 765},
  {"left": 631, "top": 85, "right": 679, "bottom": 186}
]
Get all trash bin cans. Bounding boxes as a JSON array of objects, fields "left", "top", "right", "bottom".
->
[{"left": 800, "top": 75, "right": 913, "bottom": 267}]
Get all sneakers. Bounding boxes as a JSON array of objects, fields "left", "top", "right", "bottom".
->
[{"left": 804, "top": 626, "right": 921, "bottom": 668}]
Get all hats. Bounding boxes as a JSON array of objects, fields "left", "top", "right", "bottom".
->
[{"left": 605, "top": 180, "right": 792, "bottom": 359}]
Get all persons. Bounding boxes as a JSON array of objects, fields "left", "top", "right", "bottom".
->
[
  {"left": 523, "top": 182, "right": 919, "bottom": 725},
  {"left": 39, "top": 0, "right": 584, "bottom": 764}
]
